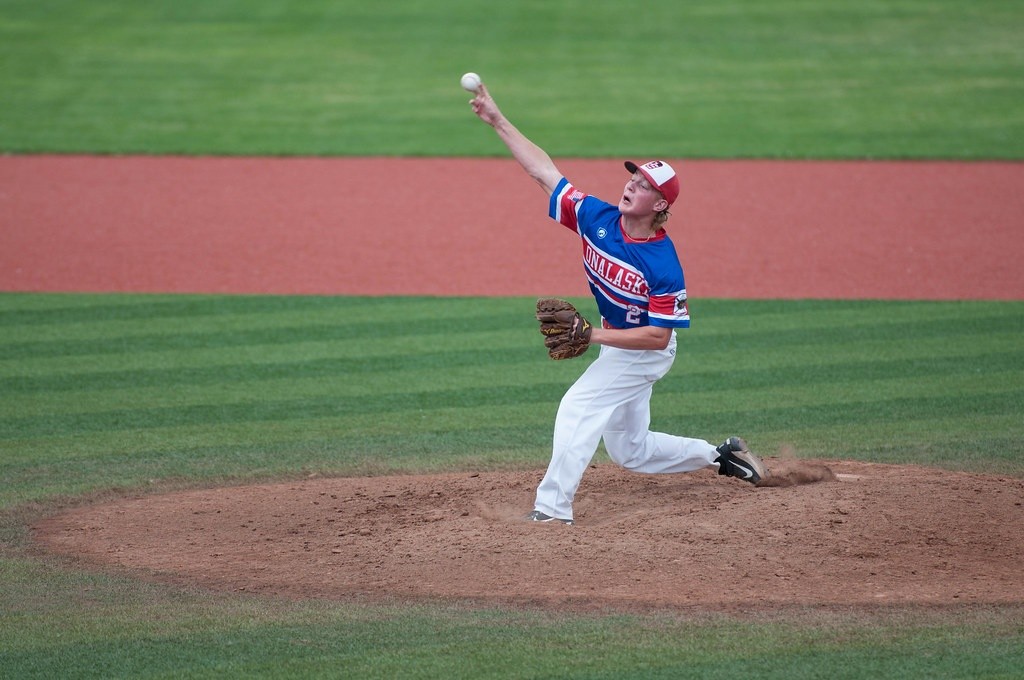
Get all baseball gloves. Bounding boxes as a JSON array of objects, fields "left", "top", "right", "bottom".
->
[{"left": 538, "top": 298, "right": 594, "bottom": 361}]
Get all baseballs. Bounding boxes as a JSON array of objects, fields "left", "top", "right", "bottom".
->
[{"left": 457, "top": 72, "right": 483, "bottom": 92}]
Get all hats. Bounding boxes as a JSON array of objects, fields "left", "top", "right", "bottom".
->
[{"left": 623, "top": 158, "right": 680, "bottom": 209}]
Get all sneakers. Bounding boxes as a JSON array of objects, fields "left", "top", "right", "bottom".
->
[
  {"left": 716, "top": 436, "right": 765, "bottom": 485},
  {"left": 515, "top": 510, "right": 574, "bottom": 526}
]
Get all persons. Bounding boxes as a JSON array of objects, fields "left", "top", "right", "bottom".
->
[{"left": 466, "top": 85, "right": 768, "bottom": 525}]
